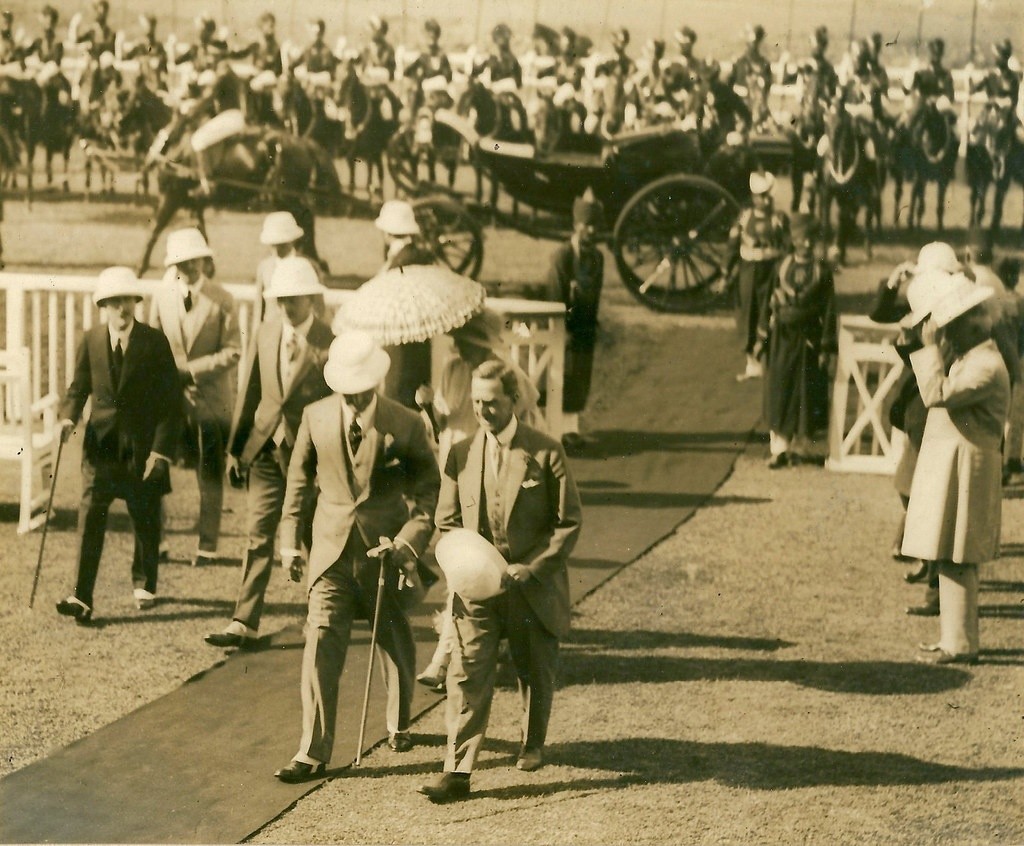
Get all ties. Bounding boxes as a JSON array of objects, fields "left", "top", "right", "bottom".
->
[
  {"left": 285, "top": 335, "right": 301, "bottom": 385},
  {"left": 495, "top": 444, "right": 505, "bottom": 477},
  {"left": 185, "top": 290, "right": 191, "bottom": 312},
  {"left": 112, "top": 339, "right": 122, "bottom": 390},
  {"left": 348, "top": 421, "right": 358, "bottom": 453}
]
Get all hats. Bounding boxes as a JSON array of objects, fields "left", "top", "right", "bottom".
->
[
  {"left": 323, "top": 333, "right": 390, "bottom": 396},
  {"left": 258, "top": 210, "right": 306, "bottom": 244},
  {"left": 164, "top": 228, "right": 214, "bottom": 266},
  {"left": 899, "top": 240, "right": 994, "bottom": 332},
  {"left": 263, "top": 258, "right": 327, "bottom": 298},
  {"left": 435, "top": 529, "right": 510, "bottom": 604},
  {"left": 92, "top": 266, "right": 144, "bottom": 307},
  {"left": 374, "top": 201, "right": 418, "bottom": 234}
]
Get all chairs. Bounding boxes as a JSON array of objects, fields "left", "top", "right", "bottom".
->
[{"left": 0, "top": 346, "right": 59, "bottom": 533}]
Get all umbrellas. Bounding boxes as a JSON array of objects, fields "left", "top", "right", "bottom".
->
[{"left": 332, "top": 263, "right": 486, "bottom": 347}]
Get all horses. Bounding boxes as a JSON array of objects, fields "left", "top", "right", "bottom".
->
[
  {"left": 890, "top": 95, "right": 958, "bottom": 231},
  {"left": 270, "top": 56, "right": 331, "bottom": 149},
  {"left": 457, "top": 59, "right": 827, "bottom": 218},
  {"left": 333, "top": 55, "right": 401, "bottom": 195},
  {"left": 0, "top": 64, "right": 270, "bottom": 192},
  {"left": 811, "top": 89, "right": 886, "bottom": 266},
  {"left": 402, "top": 68, "right": 450, "bottom": 190},
  {"left": 133, "top": 69, "right": 339, "bottom": 280},
  {"left": 967, "top": 91, "right": 1020, "bottom": 261}
]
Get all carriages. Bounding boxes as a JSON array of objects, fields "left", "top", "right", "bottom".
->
[{"left": 79, "top": 73, "right": 804, "bottom": 314}]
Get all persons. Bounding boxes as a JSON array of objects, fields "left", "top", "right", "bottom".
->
[
  {"left": 415, "top": 362, "right": 582, "bottom": 800},
  {"left": 757, "top": 212, "right": 838, "bottom": 468},
  {"left": 55, "top": 265, "right": 185, "bottom": 620},
  {"left": 534, "top": 193, "right": 603, "bottom": 449},
  {"left": 867, "top": 231, "right": 1024, "bottom": 666},
  {"left": 150, "top": 196, "right": 545, "bottom": 691},
  {"left": 718, "top": 172, "right": 794, "bottom": 381},
  {"left": 274, "top": 331, "right": 443, "bottom": 782},
  {"left": 205, "top": 257, "right": 335, "bottom": 652},
  {"left": 0, "top": 0, "right": 1024, "bottom": 155}
]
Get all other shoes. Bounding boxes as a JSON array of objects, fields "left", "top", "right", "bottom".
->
[
  {"left": 134, "top": 593, "right": 152, "bottom": 611},
  {"left": 416, "top": 674, "right": 448, "bottom": 696},
  {"left": 56, "top": 601, "right": 90, "bottom": 624}
]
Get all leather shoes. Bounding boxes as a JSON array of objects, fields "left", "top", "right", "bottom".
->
[
  {"left": 895, "top": 546, "right": 978, "bottom": 666},
  {"left": 205, "top": 633, "right": 257, "bottom": 650},
  {"left": 516, "top": 743, "right": 542, "bottom": 772},
  {"left": 418, "top": 772, "right": 469, "bottom": 800},
  {"left": 389, "top": 729, "right": 412, "bottom": 753},
  {"left": 274, "top": 760, "right": 327, "bottom": 783}
]
